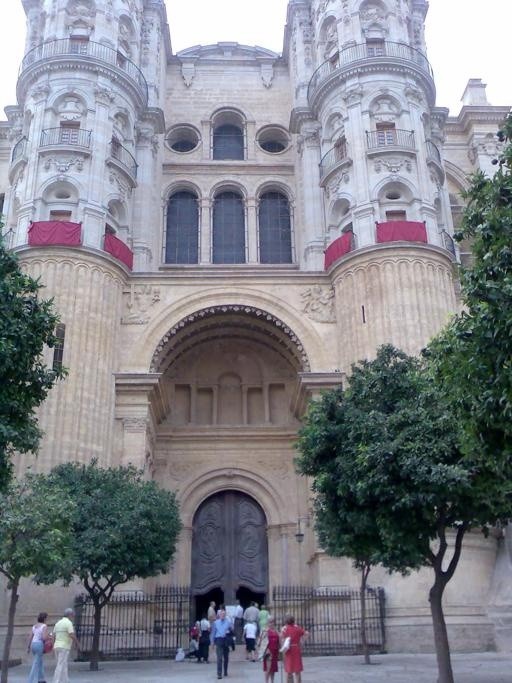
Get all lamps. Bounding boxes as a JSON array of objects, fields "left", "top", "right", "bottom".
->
[{"left": 296, "top": 515, "right": 310, "bottom": 542}]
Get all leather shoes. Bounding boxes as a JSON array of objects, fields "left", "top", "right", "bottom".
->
[{"left": 218, "top": 673, "right": 222, "bottom": 679}]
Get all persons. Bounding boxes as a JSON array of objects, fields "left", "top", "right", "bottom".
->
[
  {"left": 256, "top": 616, "right": 280, "bottom": 682},
  {"left": 26, "top": 611, "right": 53, "bottom": 682},
  {"left": 210, "top": 607, "right": 234, "bottom": 679},
  {"left": 52, "top": 606, "right": 80, "bottom": 682},
  {"left": 279, "top": 612, "right": 310, "bottom": 682},
  {"left": 189, "top": 597, "right": 270, "bottom": 663}
]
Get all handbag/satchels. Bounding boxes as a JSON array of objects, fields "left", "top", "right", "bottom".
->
[
  {"left": 44, "top": 640, "right": 53, "bottom": 653},
  {"left": 279, "top": 637, "right": 291, "bottom": 654}
]
